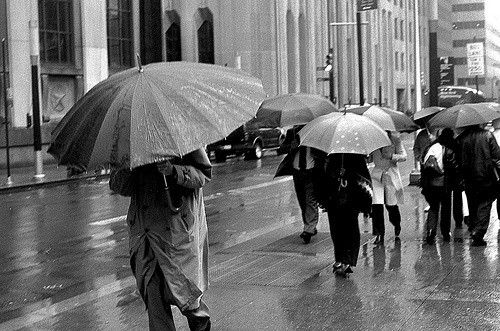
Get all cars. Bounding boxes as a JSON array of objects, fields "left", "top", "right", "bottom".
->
[{"left": 206, "top": 121, "right": 284, "bottom": 163}]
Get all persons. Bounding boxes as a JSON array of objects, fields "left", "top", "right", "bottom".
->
[
  {"left": 311, "top": 154, "right": 373, "bottom": 275},
  {"left": 365, "top": 131, "right": 407, "bottom": 245},
  {"left": 413, "top": 119, "right": 500, "bottom": 245},
  {"left": 273, "top": 125, "right": 318, "bottom": 244},
  {"left": 109, "top": 148, "right": 212, "bottom": 331}
]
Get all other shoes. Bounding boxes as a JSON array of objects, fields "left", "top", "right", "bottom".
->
[
  {"left": 373, "top": 235, "right": 384, "bottom": 244},
  {"left": 300, "top": 228, "right": 317, "bottom": 244},
  {"left": 333, "top": 262, "right": 354, "bottom": 274},
  {"left": 426, "top": 228, "right": 436, "bottom": 246},
  {"left": 424, "top": 205, "right": 430, "bottom": 211},
  {"left": 395, "top": 223, "right": 401, "bottom": 237},
  {"left": 469, "top": 230, "right": 487, "bottom": 246},
  {"left": 443, "top": 233, "right": 451, "bottom": 241}
]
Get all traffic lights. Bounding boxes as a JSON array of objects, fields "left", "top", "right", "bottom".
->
[{"left": 326, "top": 50, "right": 333, "bottom": 72}]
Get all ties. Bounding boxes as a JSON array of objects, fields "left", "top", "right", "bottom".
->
[{"left": 298, "top": 146, "right": 307, "bottom": 169}]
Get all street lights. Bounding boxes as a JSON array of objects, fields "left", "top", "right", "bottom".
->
[{"left": 327, "top": 22, "right": 371, "bottom": 102}]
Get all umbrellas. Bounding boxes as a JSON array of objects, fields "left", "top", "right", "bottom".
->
[
  {"left": 255, "top": 92, "right": 341, "bottom": 133},
  {"left": 297, "top": 107, "right": 393, "bottom": 156},
  {"left": 399, "top": 106, "right": 446, "bottom": 134},
  {"left": 427, "top": 102, "right": 500, "bottom": 130},
  {"left": 47, "top": 56, "right": 265, "bottom": 212},
  {"left": 341, "top": 104, "right": 420, "bottom": 133}
]
